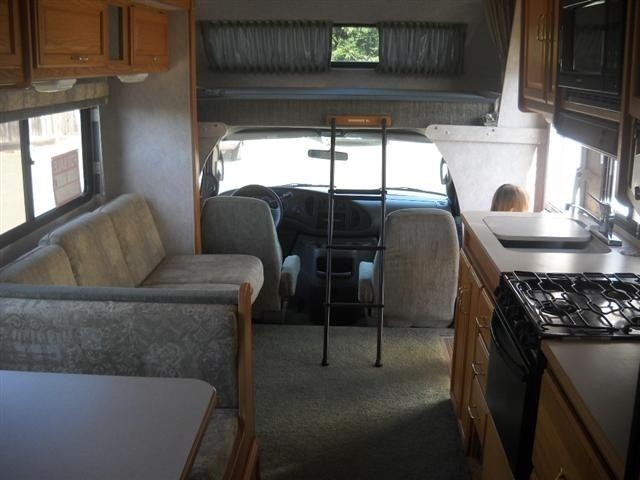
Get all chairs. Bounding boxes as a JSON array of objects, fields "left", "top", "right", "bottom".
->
[{"left": 200, "top": 196, "right": 467, "bottom": 328}]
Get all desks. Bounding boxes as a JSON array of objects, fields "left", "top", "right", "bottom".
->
[{"left": 0, "top": 369, "right": 218, "bottom": 479}]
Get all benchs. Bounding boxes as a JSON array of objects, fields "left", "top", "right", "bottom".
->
[
  {"left": 0, "top": 281, "right": 260, "bottom": 479},
  {"left": 3, "top": 195, "right": 262, "bottom": 312}
]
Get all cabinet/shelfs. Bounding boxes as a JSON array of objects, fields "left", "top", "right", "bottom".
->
[
  {"left": 479, "top": 324, "right": 639, "bottom": 479},
  {"left": 0, "top": 0, "right": 168, "bottom": 91},
  {"left": 616, "top": 2, "right": 640, "bottom": 210},
  {"left": 451, "top": 209, "right": 630, "bottom": 465},
  {"left": 517, "top": 0, "right": 554, "bottom": 116}
]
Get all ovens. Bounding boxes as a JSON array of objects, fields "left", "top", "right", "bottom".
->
[{"left": 485, "top": 274, "right": 547, "bottom": 480}]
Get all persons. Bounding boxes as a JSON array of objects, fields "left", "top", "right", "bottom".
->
[{"left": 490, "top": 182, "right": 530, "bottom": 211}]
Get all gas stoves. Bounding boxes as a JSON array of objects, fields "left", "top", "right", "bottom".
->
[{"left": 501, "top": 269, "right": 640, "bottom": 345}]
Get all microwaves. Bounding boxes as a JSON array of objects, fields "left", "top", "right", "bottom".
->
[{"left": 557, "top": 0, "right": 628, "bottom": 94}]
